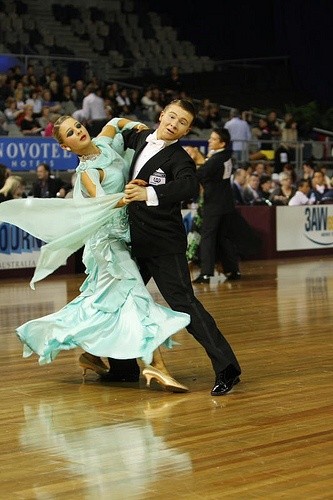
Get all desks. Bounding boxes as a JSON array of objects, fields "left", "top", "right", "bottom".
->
[{"left": 238, "top": 206, "right": 333, "bottom": 261}]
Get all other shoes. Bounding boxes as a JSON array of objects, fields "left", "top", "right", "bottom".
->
[
  {"left": 227, "top": 271, "right": 241, "bottom": 280},
  {"left": 192, "top": 274, "right": 210, "bottom": 284}
]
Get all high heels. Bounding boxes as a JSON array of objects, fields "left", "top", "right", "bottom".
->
[
  {"left": 142, "top": 364, "right": 189, "bottom": 393},
  {"left": 78, "top": 352, "right": 110, "bottom": 377}
]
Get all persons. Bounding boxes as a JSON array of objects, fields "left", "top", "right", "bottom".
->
[
  {"left": 0, "top": 164, "right": 27, "bottom": 202},
  {"left": 0, "top": 66, "right": 225, "bottom": 137},
  {"left": 29, "top": 164, "right": 72, "bottom": 199},
  {"left": 191, "top": 127, "right": 240, "bottom": 285},
  {"left": 0, "top": 116, "right": 191, "bottom": 393},
  {"left": 94, "top": 99, "right": 241, "bottom": 396},
  {"left": 225, "top": 109, "right": 252, "bottom": 170},
  {"left": 82, "top": 84, "right": 111, "bottom": 137},
  {"left": 232, "top": 110, "right": 333, "bottom": 207}
]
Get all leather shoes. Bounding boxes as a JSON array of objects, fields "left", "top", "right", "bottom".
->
[
  {"left": 100, "top": 372, "right": 139, "bottom": 382},
  {"left": 211, "top": 364, "right": 242, "bottom": 396}
]
